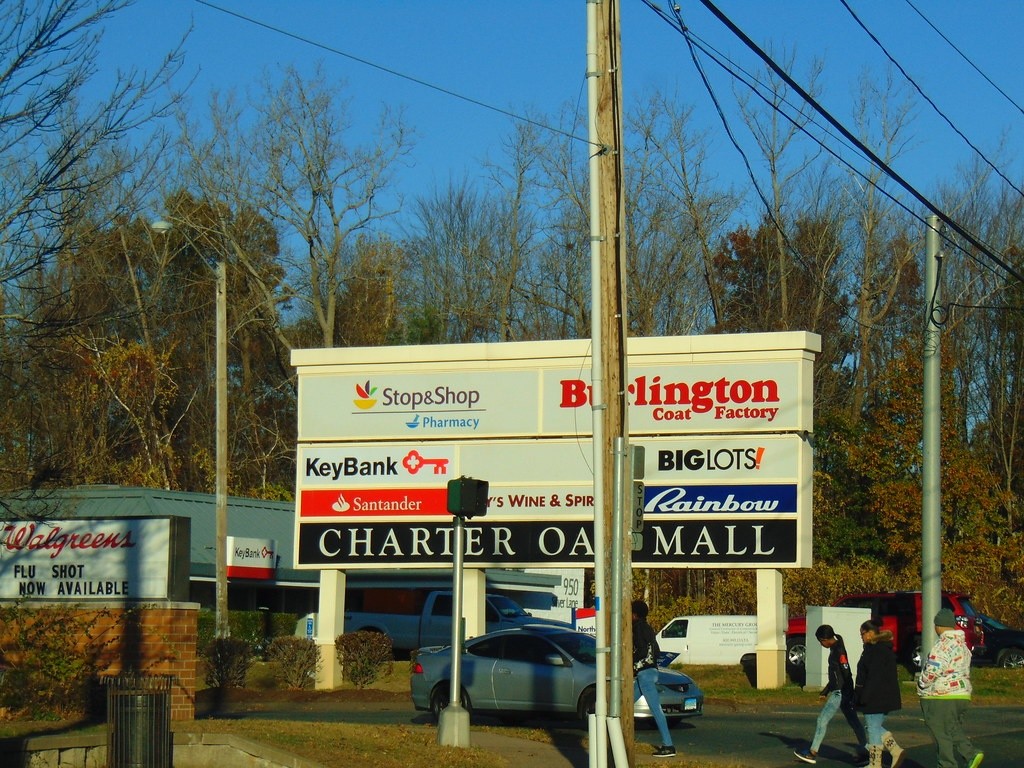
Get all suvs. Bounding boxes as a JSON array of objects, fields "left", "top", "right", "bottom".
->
[{"left": 786, "top": 590, "right": 987, "bottom": 682}]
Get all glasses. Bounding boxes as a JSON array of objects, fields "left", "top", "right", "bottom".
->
[{"left": 859, "top": 629, "right": 871, "bottom": 635}]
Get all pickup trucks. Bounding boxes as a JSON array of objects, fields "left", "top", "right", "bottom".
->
[
  {"left": 345, "top": 591, "right": 576, "bottom": 650},
  {"left": 492, "top": 584, "right": 597, "bottom": 636}
]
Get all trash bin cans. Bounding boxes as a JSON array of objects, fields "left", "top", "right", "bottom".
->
[{"left": 104, "top": 674, "right": 171, "bottom": 768}]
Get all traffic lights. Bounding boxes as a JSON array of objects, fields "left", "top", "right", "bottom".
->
[{"left": 462, "top": 477, "right": 489, "bottom": 519}]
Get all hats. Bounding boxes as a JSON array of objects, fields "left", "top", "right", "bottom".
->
[
  {"left": 933, "top": 608, "right": 957, "bottom": 628},
  {"left": 630, "top": 599, "right": 649, "bottom": 617}
]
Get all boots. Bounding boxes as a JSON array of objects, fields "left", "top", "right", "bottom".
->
[
  {"left": 880, "top": 730, "right": 907, "bottom": 768},
  {"left": 863, "top": 745, "right": 884, "bottom": 768}
]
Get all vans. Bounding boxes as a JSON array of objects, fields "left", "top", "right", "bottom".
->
[{"left": 655, "top": 615, "right": 757, "bottom": 665}]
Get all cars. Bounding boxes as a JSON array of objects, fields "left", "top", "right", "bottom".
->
[
  {"left": 410, "top": 624, "right": 705, "bottom": 732},
  {"left": 976, "top": 613, "right": 1024, "bottom": 670}
]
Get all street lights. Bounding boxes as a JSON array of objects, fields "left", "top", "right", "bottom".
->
[{"left": 153, "top": 221, "right": 228, "bottom": 639}]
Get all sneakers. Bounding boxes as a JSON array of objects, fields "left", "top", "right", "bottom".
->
[
  {"left": 968, "top": 750, "right": 984, "bottom": 768},
  {"left": 793, "top": 748, "right": 817, "bottom": 764},
  {"left": 651, "top": 746, "right": 676, "bottom": 758}
]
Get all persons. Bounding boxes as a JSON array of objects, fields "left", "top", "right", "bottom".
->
[
  {"left": 793, "top": 625, "right": 871, "bottom": 764},
  {"left": 915, "top": 608, "right": 984, "bottom": 768},
  {"left": 631, "top": 599, "right": 677, "bottom": 757},
  {"left": 845, "top": 616, "right": 907, "bottom": 768}
]
